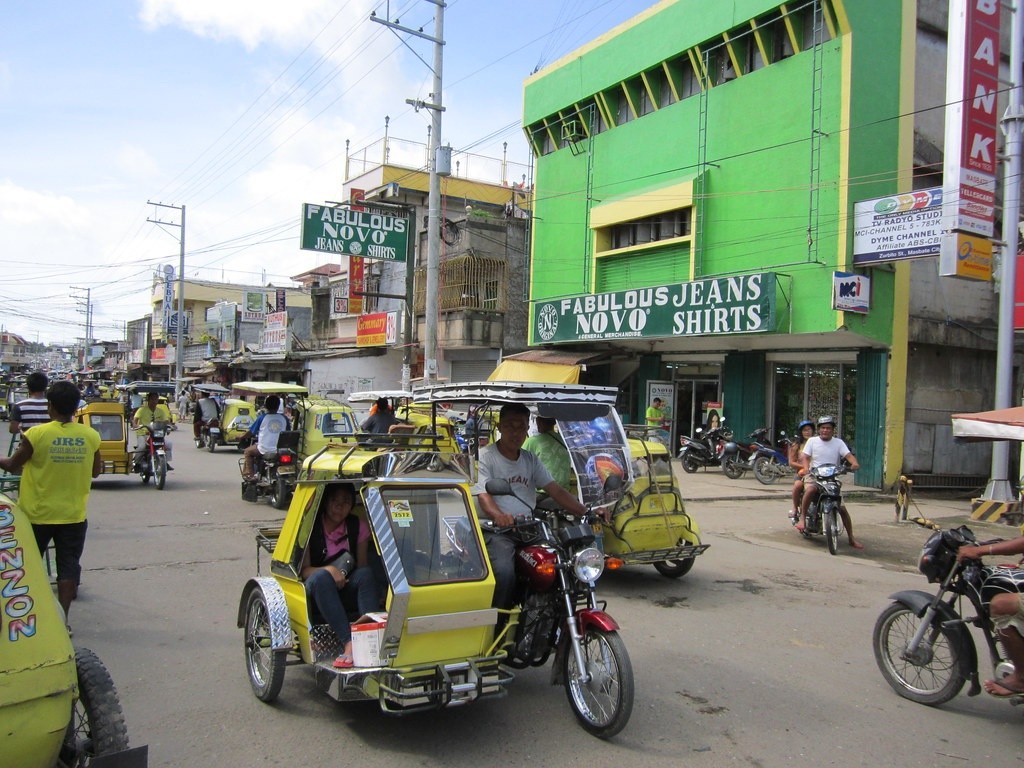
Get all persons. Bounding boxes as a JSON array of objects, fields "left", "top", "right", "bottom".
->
[
  {"left": 522, "top": 415, "right": 570, "bottom": 497},
  {"left": 788, "top": 419, "right": 818, "bottom": 517},
  {"left": 464, "top": 403, "right": 481, "bottom": 434},
  {"left": 360, "top": 397, "right": 398, "bottom": 452},
  {"left": 130, "top": 391, "right": 176, "bottom": 472},
  {"left": 117, "top": 389, "right": 143, "bottom": 424},
  {"left": 958, "top": 531, "right": 1024, "bottom": 698},
  {"left": 237, "top": 395, "right": 291, "bottom": 481},
  {"left": 193, "top": 392, "right": 232, "bottom": 441},
  {"left": 795, "top": 414, "right": 866, "bottom": 549},
  {"left": 299, "top": 483, "right": 381, "bottom": 671},
  {"left": 470, "top": 401, "right": 613, "bottom": 657},
  {"left": 646, "top": 398, "right": 666, "bottom": 440},
  {"left": 73, "top": 381, "right": 126, "bottom": 408},
  {"left": 706, "top": 409, "right": 720, "bottom": 432},
  {"left": 9, "top": 373, "right": 54, "bottom": 447},
  {"left": 177, "top": 390, "right": 189, "bottom": 421},
  {"left": 0, "top": 381, "right": 102, "bottom": 638}
]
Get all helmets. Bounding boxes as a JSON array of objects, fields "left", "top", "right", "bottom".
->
[
  {"left": 817, "top": 415, "right": 836, "bottom": 428},
  {"left": 798, "top": 419, "right": 816, "bottom": 432}
]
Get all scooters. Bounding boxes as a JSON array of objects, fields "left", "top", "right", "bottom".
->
[
  {"left": 678, "top": 417, "right": 734, "bottom": 474},
  {"left": 747, "top": 430, "right": 798, "bottom": 485},
  {"left": 716, "top": 426, "right": 773, "bottom": 480}
]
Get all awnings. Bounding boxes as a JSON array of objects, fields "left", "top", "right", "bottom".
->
[
  {"left": 187, "top": 367, "right": 217, "bottom": 377},
  {"left": 507, "top": 348, "right": 608, "bottom": 370}
]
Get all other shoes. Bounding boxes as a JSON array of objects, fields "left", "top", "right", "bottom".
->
[
  {"left": 788, "top": 507, "right": 798, "bottom": 518},
  {"left": 796, "top": 521, "right": 806, "bottom": 531},
  {"left": 849, "top": 542, "right": 865, "bottom": 549}
]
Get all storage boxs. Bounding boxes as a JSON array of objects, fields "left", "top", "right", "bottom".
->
[{"left": 350, "top": 612, "right": 390, "bottom": 667}]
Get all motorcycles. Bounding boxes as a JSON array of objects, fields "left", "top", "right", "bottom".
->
[{"left": 791, "top": 460, "right": 855, "bottom": 555}]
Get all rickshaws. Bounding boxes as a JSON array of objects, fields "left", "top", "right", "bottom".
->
[
  {"left": 238, "top": 381, "right": 637, "bottom": 740},
  {"left": 873, "top": 408, "right": 1023, "bottom": 710},
  {"left": 76, "top": 382, "right": 176, "bottom": 490},
  {"left": 0, "top": 486, "right": 150, "bottom": 768},
  {"left": 0, "top": 372, "right": 178, "bottom": 432},
  {"left": 190, "top": 384, "right": 710, "bottom": 579}
]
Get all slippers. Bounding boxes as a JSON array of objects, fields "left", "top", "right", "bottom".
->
[
  {"left": 333, "top": 654, "right": 354, "bottom": 668},
  {"left": 983, "top": 677, "right": 1024, "bottom": 698}
]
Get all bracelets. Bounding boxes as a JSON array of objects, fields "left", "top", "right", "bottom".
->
[
  {"left": 584, "top": 508, "right": 590, "bottom": 514},
  {"left": 989, "top": 544, "right": 993, "bottom": 555}
]
